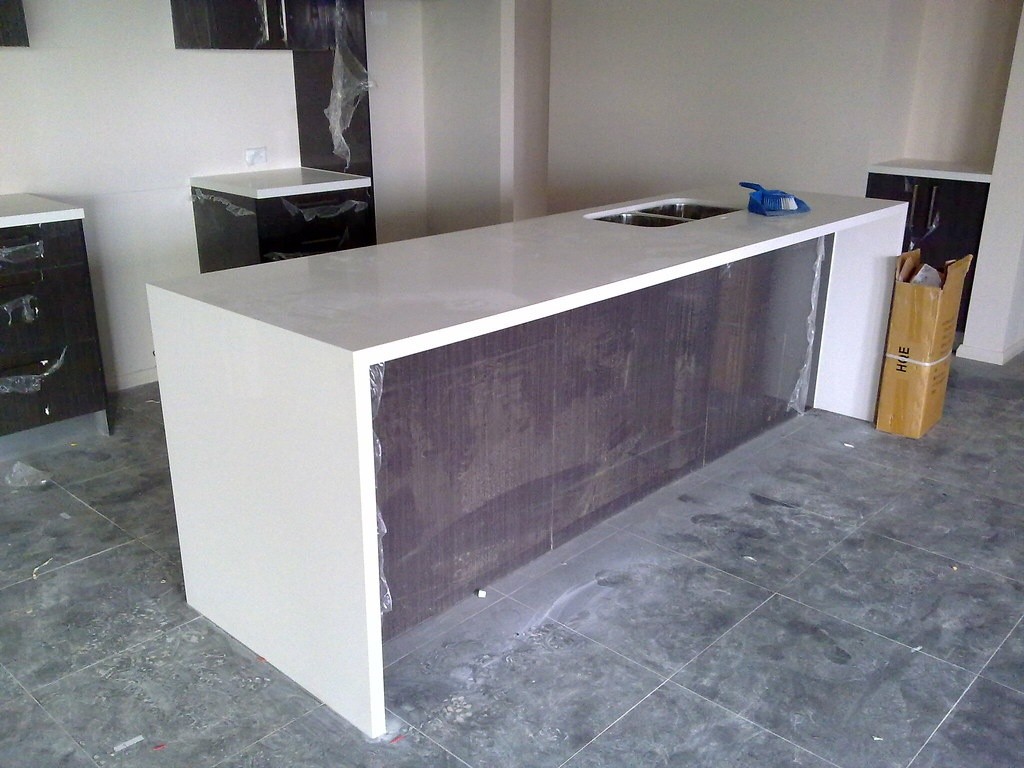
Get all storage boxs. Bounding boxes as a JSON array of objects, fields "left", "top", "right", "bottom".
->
[{"left": 873, "top": 250, "right": 973, "bottom": 440}]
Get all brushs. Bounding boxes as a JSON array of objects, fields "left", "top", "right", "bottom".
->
[{"left": 762, "top": 193, "right": 799, "bottom": 211}]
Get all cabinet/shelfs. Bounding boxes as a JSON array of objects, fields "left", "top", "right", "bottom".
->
[
  {"left": 0, "top": 193, "right": 112, "bottom": 462},
  {"left": 866, "top": 157, "right": 993, "bottom": 354},
  {"left": 189, "top": 166, "right": 376, "bottom": 276},
  {"left": 169, "top": 0, "right": 335, "bottom": 55}
]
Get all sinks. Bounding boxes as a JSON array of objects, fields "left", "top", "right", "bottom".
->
[
  {"left": 581, "top": 208, "right": 693, "bottom": 228},
  {"left": 636, "top": 198, "right": 745, "bottom": 220}
]
